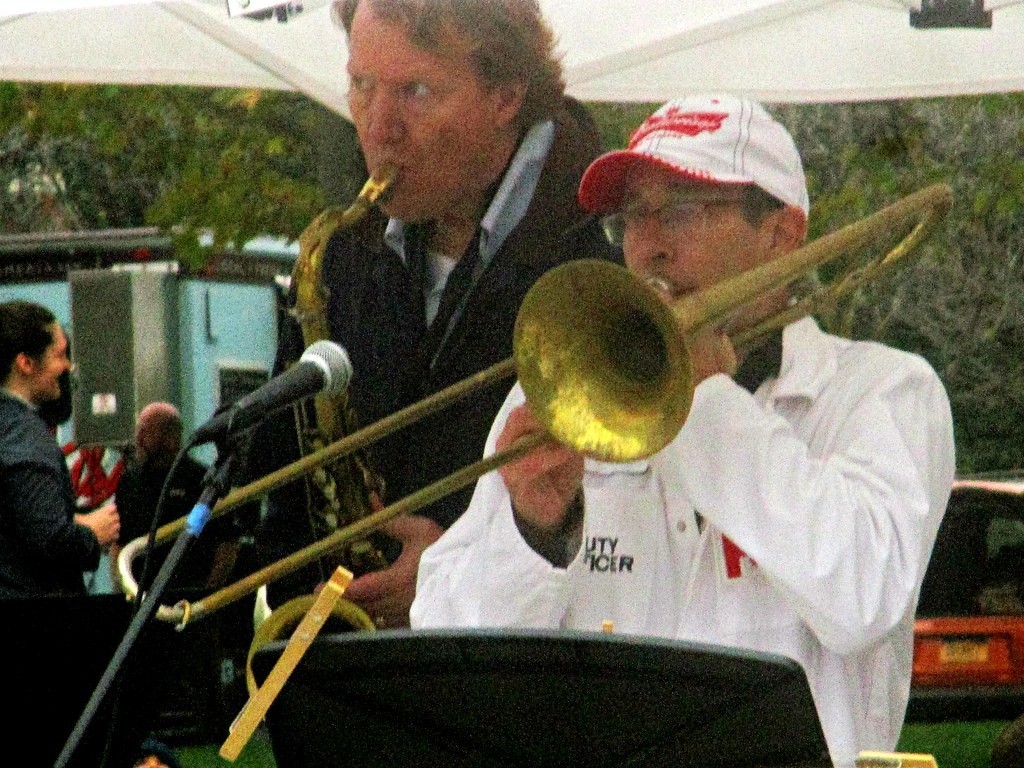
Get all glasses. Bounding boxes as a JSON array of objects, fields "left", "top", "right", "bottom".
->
[{"left": 598, "top": 198, "right": 769, "bottom": 247}]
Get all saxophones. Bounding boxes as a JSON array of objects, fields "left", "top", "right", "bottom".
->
[{"left": 245, "top": 156, "right": 401, "bottom": 721}]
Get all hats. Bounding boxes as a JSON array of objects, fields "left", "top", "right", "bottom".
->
[{"left": 579, "top": 93, "right": 810, "bottom": 235}]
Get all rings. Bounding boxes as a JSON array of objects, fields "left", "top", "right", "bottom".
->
[{"left": 376, "top": 617, "right": 385, "bottom": 628}]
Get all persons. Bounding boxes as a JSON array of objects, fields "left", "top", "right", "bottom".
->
[
  {"left": 405, "top": 86, "right": 956, "bottom": 768},
  {"left": 107, "top": 403, "right": 238, "bottom": 739},
  {"left": 0, "top": 299, "right": 120, "bottom": 768},
  {"left": 236, "top": 0, "right": 620, "bottom": 631}
]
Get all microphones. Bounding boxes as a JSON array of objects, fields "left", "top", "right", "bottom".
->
[{"left": 188, "top": 336, "right": 353, "bottom": 449}]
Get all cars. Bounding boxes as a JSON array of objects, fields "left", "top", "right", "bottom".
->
[{"left": 911, "top": 477, "right": 1024, "bottom": 692}]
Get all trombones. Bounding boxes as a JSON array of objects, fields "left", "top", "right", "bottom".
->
[{"left": 115, "top": 185, "right": 958, "bottom": 624}]
